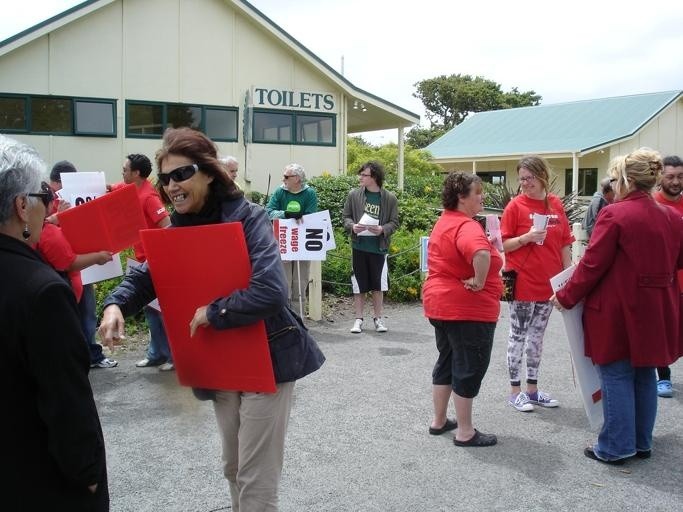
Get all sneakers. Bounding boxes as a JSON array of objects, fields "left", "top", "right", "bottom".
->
[
  {"left": 89, "top": 358, "right": 119, "bottom": 370},
  {"left": 373, "top": 316, "right": 387, "bottom": 333},
  {"left": 135, "top": 357, "right": 166, "bottom": 368},
  {"left": 160, "top": 361, "right": 174, "bottom": 372},
  {"left": 525, "top": 389, "right": 560, "bottom": 409},
  {"left": 508, "top": 392, "right": 535, "bottom": 413},
  {"left": 350, "top": 317, "right": 363, "bottom": 333}
]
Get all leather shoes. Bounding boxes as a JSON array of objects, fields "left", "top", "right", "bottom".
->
[
  {"left": 635, "top": 449, "right": 652, "bottom": 458},
  {"left": 583, "top": 446, "right": 627, "bottom": 466}
]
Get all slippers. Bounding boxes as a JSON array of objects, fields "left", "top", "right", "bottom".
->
[
  {"left": 453, "top": 427, "right": 497, "bottom": 447},
  {"left": 429, "top": 417, "right": 458, "bottom": 436}
]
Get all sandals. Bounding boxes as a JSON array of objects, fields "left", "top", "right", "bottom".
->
[{"left": 656, "top": 378, "right": 673, "bottom": 398}]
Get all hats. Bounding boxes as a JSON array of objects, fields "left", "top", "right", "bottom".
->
[{"left": 49, "top": 160, "right": 77, "bottom": 184}]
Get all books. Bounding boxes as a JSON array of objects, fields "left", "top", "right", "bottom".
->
[{"left": 531, "top": 213, "right": 550, "bottom": 246}]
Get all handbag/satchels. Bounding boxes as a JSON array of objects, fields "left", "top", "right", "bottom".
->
[{"left": 498, "top": 270, "right": 517, "bottom": 302}]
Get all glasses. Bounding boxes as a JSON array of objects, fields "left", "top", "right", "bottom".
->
[
  {"left": 516, "top": 175, "right": 539, "bottom": 183},
  {"left": 156, "top": 163, "right": 204, "bottom": 187},
  {"left": 25, "top": 188, "right": 49, "bottom": 209},
  {"left": 284, "top": 175, "right": 296, "bottom": 179}
]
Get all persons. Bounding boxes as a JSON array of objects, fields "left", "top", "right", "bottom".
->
[
  {"left": 46, "top": 160, "right": 118, "bottom": 367},
  {"left": 422, "top": 171, "right": 504, "bottom": 447},
  {"left": 98, "top": 127, "right": 325, "bottom": 512},
  {"left": 649, "top": 155, "right": 683, "bottom": 397},
  {"left": 500, "top": 156, "right": 576, "bottom": 412},
  {"left": 342, "top": 161, "right": 398, "bottom": 333},
  {"left": 0, "top": 135, "right": 109, "bottom": 508},
  {"left": 106, "top": 153, "right": 174, "bottom": 371},
  {"left": 265, "top": 163, "right": 318, "bottom": 316},
  {"left": 31, "top": 181, "right": 113, "bottom": 304},
  {"left": 583, "top": 176, "right": 616, "bottom": 236},
  {"left": 548, "top": 147, "right": 683, "bottom": 466},
  {"left": 220, "top": 156, "right": 239, "bottom": 181}
]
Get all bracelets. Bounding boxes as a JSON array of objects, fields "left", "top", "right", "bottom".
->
[
  {"left": 517, "top": 236, "right": 527, "bottom": 247},
  {"left": 473, "top": 275, "right": 478, "bottom": 286}
]
